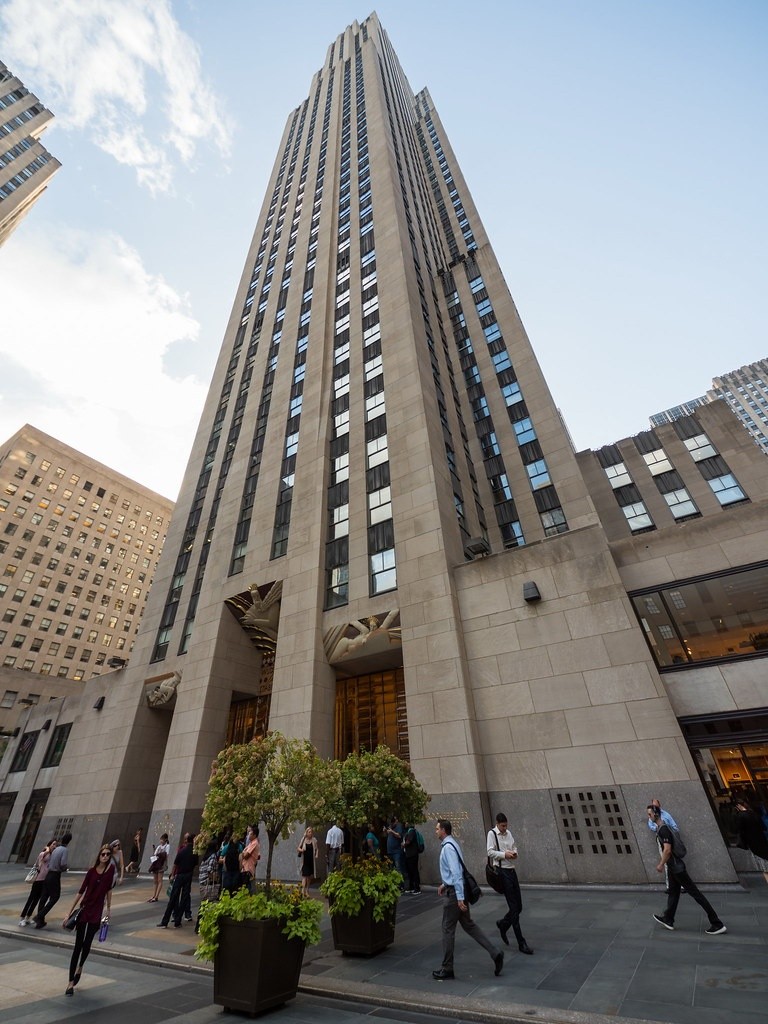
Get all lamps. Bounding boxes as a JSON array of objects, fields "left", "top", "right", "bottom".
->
[
  {"left": 107, "top": 657, "right": 128, "bottom": 668},
  {"left": 523, "top": 581, "right": 542, "bottom": 604},
  {"left": 13, "top": 727, "right": 20, "bottom": 736},
  {"left": 93, "top": 696, "right": 105, "bottom": 710},
  {"left": 42, "top": 719, "right": 52, "bottom": 730}
]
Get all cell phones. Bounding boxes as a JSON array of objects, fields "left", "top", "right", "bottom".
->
[{"left": 513, "top": 853, "right": 517, "bottom": 855}]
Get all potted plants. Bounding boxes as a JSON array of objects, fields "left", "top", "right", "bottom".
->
[
  {"left": 321, "top": 743, "right": 431, "bottom": 967},
  {"left": 192, "top": 730, "right": 343, "bottom": 1017}
]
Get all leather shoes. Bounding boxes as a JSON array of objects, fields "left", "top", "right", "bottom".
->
[
  {"left": 497, "top": 919, "right": 509, "bottom": 945},
  {"left": 494, "top": 951, "right": 504, "bottom": 976},
  {"left": 519, "top": 944, "right": 533, "bottom": 954},
  {"left": 432, "top": 968, "right": 454, "bottom": 979}
]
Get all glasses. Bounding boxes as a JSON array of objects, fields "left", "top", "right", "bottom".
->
[
  {"left": 114, "top": 844, "right": 120, "bottom": 847},
  {"left": 100, "top": 853, "right": 111, "bottom": 857}
]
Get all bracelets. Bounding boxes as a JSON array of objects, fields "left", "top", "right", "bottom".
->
[{"left": 442, "top": 884, "right": 446, "bottom": 889}]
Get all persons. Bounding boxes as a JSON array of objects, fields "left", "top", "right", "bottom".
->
[
  {"left": 487, "top": 813, "right": 533, "bottom": 954},
  {"left": 365, "top": 815, "right": 422, "bottom": 895},
  {"left": 18, "top": 833, "right": 72, "bottom": 929},
  {"left": 329, "top": 616, "right": 381, "bottom": 662},
  {"left": 240, "top": 589, "right": 279, "bottom": 641},
  {"left": 147, "top": 833, "right": 170, "bottom": 902},
  {"left": 706, "top": 763, "right": 721, "bottom": 793},
  {"left": 432, "top": 819, "right": 504, "bottom": 979},
  {"left": 153, "top": 671, "right": 181, "bottom": 706},
  {"left": 125, "top": 827, "right": 142, "bottom": 872},
  {"left": 62, "top": 839, "right": 124, "bottom": 997},
  {"left": 296, "top": 827, "right": 318, "bottom": 897},
  {"left": 156, "top": 824, "right": 261, "bottom": 935},
  {"left": 647, "top": 805, "right": 727, "bottom": 934},
  {"left": 648, "top": 798, "right": 686, "bottom": 893},
  {"left": 325, "top": 821, "right": 344, "bottom": 878}
]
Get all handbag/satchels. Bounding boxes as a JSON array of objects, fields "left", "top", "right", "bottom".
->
[
  {"left": 486, "top": 864, "right": 505, "bottom": 895},
  {"left": 200, "top": 877, "right": 220, "bottom": 901},
  {"left": 25, "top": 867, "right": 38, "bottom": 882},
  {"left": 99, "top": 918, "right": 108, "bottom": 942},
  {"left": 464, "top": 871, "right": 482, "bottom": 905},
  {"left": 64, "top": 909, "right": 78, "bottom": 932},
  {"left": 297, "top": 836, "right": 306, "bottom": 857}
]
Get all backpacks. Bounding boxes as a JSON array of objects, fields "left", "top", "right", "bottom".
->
[
  {"left": 408, "top": 828, "right": 425, "bottom": 853},
  {"left": 656, "top": 824, "right": 687, "bottom": 857}
]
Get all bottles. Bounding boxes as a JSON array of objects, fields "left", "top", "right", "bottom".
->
[{"left": 99, "top": 924, "right": 108, "bottom": 942}]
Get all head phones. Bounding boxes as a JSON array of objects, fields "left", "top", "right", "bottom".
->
[{"left": 652, "top": 804, "right": 659, "bottom": 820}]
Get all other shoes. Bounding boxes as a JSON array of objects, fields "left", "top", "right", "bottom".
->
[
  {"left": 401, "top": 889, "right": 414, "bottom": 893},
  {"left": 20, "top": 917, "right": 30, "bottom": 926},
  {"left": 156, "top": 924, "right": 166, "bottom": 929},
  {"left": 653, "top": 915, "right": 674, "bottom": 930},
  {"left": 32, "top": 916, "right": 47, "bottom": 929},
  {"left": 125, "top": 867, "right": 128, "bottom": 872},
  {"left": 174, "top": 923, "right": 182, "bottom": 928},
  {"left": 411, "top": 890, "right": 421, "bottom": 895},
  {"left": 705, "top": 924, "right": 727, "bottom": 934},
  {"left": 73, "top": 967, "right": 82, "bottom": 986},
  {"left": 65, "top": 987, "right": 73, "bottom": 996}
]
